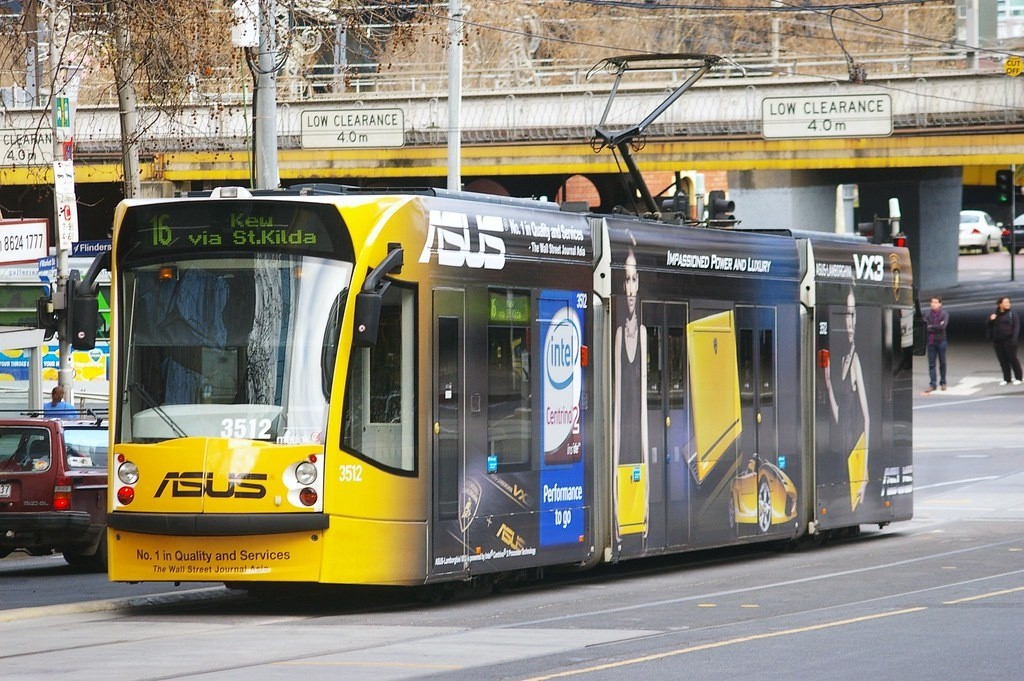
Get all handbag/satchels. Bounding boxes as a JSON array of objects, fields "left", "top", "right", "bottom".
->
[{"left": 985, "top": 325, "right": 992, "bottom": 341}]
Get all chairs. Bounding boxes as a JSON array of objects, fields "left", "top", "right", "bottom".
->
[{"left": 29, "top": 440, "right": 50, "bottom": 459}]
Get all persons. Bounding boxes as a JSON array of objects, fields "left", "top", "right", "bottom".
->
[
  {"left": 43, "top": 387, "right": 80, "bottom": 419},
  {"left": 615, "top": 229, "right": 650, "bottom": 555},
  {"left": 923, "top": 296, "right": 950, "bottom": 392},
  {"left": 986, "top": 296, "right": 1023, "bottom": 386},
  {"left": 825, "top": 275, "right": 870, "bottom": 513}
]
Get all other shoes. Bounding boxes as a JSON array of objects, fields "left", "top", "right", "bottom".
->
[
  {"left": 1013, "top": 379, "right": 1022, "bottom": 385},
  {"left": 926, "top": 386, "right": 936, "bottom": 392},
  {"left": 1000, "top": 380, "right": 1009, "bottom": 386},
  {"left": 941, "top": 384, "right": 946, "bottom": 390}
]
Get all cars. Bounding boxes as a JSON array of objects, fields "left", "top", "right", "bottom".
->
[
  {"left": 1000, "top": 213, "right": 1024, "bottom": 255},
  {"left": 957, "top": 210, "right": 1004, "bottom": 254}
]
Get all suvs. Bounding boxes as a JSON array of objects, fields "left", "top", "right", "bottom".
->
[{"left": 0, "top": 408, "right": 109, "bottom": 574}]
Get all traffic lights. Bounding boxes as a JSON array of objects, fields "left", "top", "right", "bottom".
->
[
  {"left": 64, "top": 52, "right": 931, "bottom": 605},
  {"left": 995, "top": 169, "right": 1013, "bottom": 206},
  {"left": 707, "top": 189, "right": 736, "bottom": 227}
]
